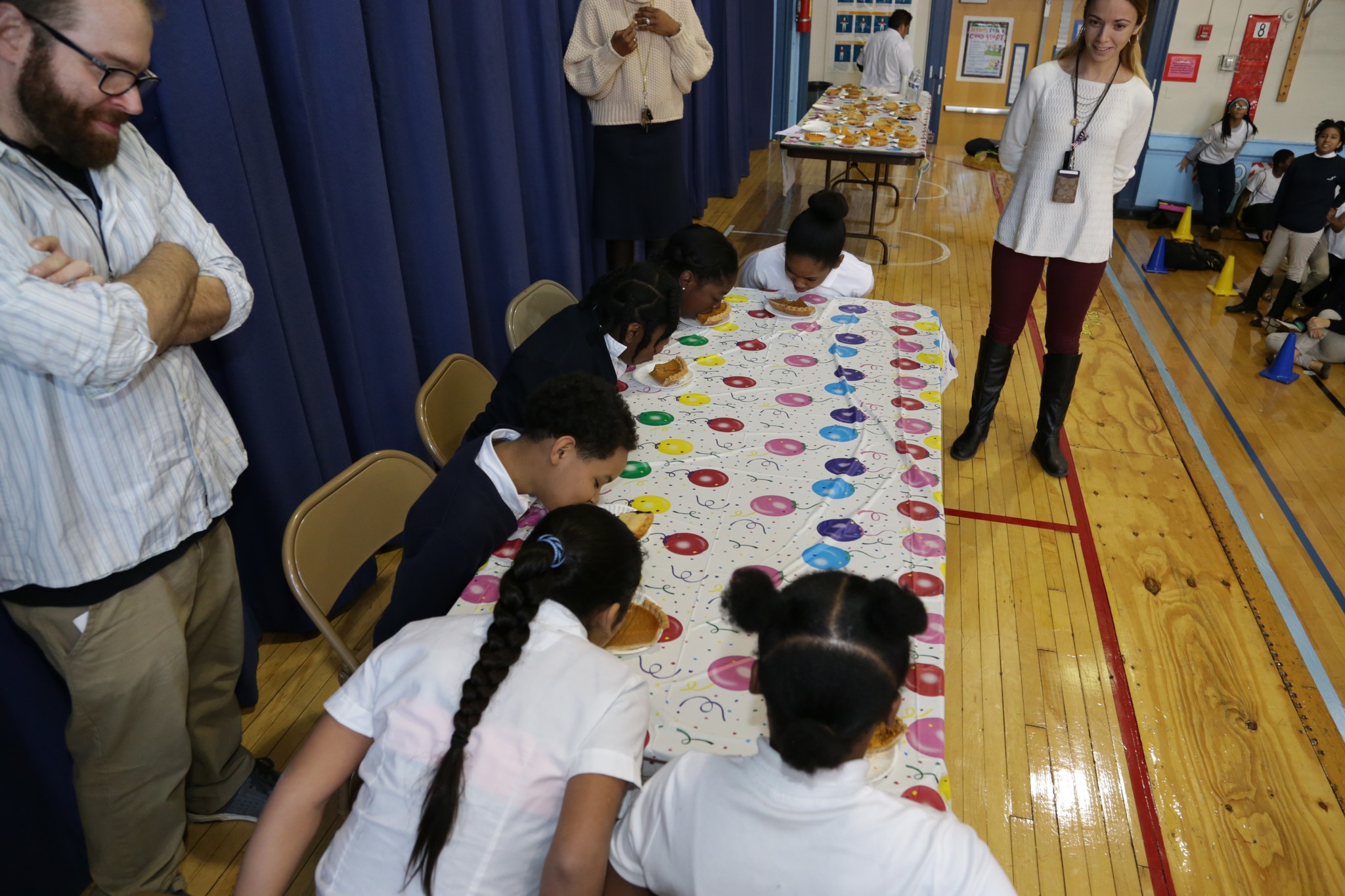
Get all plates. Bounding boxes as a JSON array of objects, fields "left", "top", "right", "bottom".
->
[
  {"left": 596, "top": 504, "right": 651, "bottom": 541},
  {"left": 864, "top": 743, "right": 895, "bottom": 781},
  {"left": 678, "top": 314, "right": 731, "bottom": 328},
  {"left": 764, "top": 300, "right": 818, "bottom": 319},
  {"left": 633, "top": 361, "right": 694, "bottom": 388},
  {"left": 802, "top": 85, "right": 922, "bottom": 150},
  {"left": 602, "top": 590, "right": 663, "bottom": 655}
]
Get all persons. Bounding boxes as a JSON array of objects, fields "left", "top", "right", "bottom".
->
[
  {"left": 1176, "top": 96, "right": 1259, "bottom": 241},
  {"left": 236, "top": 503, "right": 649, "bottom": 896},
  {"left": 605, "top": 565, "right": 1017, "bottom": 896},
  {"left": 741, "top": 190, "right": 875, "bottom": 298},
  {"left": 857, "top": 9, "right": 915, "bottom": 94},
  {"left": 0, "top": 1, "right": 280, "bottom": 896},
  {"left": 948, "top": 1, "right": 1153, "bottom": 476},
  {"left": 371, "top": 220, "right": 740, "bottom": 649},
  {"left": 1230, "top": 118, "right": 1345, "bottom": 378},
  {"left": 564, "top": 0, "right": 715, "bottom": 269}
]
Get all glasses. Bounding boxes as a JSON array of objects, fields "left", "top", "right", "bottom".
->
[
  {"left": 14, "top": 5, "right": 160, "bottom": 98},
  {"left": 1231, "top": 102, "right": 1249, "bottom": 110}
]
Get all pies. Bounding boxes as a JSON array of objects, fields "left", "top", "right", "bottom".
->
[
  {"left": 603, "top": 600, "right": 669, "bottom": 652},
  {"left": 618, "top": 512, "right": 654, "bottom": 539},
  {"left": 696, "top": 301, "right": 731, "bottom": 326},
  {"left": 804, "top": 83, "right": 922, "bottom": 148},
  {"left": 648, "top": 355, "right": 688, "bottom": 387},
  {"left": 768, "top": 298, "right": 815, "bottom": 317},
  {"left": 867, "top": 716, "right": 908, "bottom": 754}
]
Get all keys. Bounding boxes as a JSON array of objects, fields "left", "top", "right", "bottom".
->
[{"left": 641, "top": 107, "right": 651, "bottom": 132}]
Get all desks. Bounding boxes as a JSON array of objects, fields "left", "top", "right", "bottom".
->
[
  {"left": 779, "top": 87, "right": 931, "bottom": 266},
  {"left": 445, "top": 285, "right": 959, "bottom": 818}
]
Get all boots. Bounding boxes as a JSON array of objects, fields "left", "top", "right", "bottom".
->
[
  {"left": 1030, "top": 353, "right": 1083, "bottom": 478},
  {"left": 950, "top": 335, "right": 1015, "bottom": 461},
  {"left": 1250, "top": 276, "right": 1302, "bottom": 326},
  {"left": 1226, "top": 265, "right": 1274, "bottom": 313}
]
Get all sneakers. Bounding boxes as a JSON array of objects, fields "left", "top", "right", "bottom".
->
[{"left": 188, "top": 757, "right": 283, "bottom": 823}]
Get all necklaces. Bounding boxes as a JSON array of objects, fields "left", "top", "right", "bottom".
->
[{"left": 1071, "top": 66, "right": 1117, "bottom": 123}]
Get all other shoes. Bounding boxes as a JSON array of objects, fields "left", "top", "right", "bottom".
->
[
  {"left": 1210, "top": 227, "right": 1222, "bottom": 241},
  {"left": 1261, "top": 316, "right": 1301, "bottom": 335},
  {"left": 1289, "top": 299, "right": 1307, "bottom": 311},
  {"left": 1317, "top": 363, "right": 1330, "bottom": 379},
  {"left": 1264, "top": 354, "right": 1274, "bottom": 367},
  {"left": 1261, "top": 287, "right": 1271, "bottom": 302}
]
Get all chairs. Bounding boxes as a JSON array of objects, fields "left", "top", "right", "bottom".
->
[
  {"left": 283, "top": 451, "right": 437, "bottom": 672},
  {"left": 414, "top": 354, "right": 498, "bottom": 468},
  {"left": 506, "top": 278, "right": 578, "bottom": 352}
]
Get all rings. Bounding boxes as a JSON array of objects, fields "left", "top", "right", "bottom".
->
[
  {"left": 646, "top": 18, "right": 649, "bottom": 26},
  {"left": 633, "top": 39, "right": 636, "bottom": 43}
]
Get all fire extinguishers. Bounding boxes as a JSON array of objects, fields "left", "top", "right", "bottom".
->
[{"left": 796, "top": 0, "right": 812, "bottom": 32}]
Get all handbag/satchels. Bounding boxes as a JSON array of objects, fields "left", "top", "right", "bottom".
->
[
  {"left": 1146, "top": 208, "right": 1183, "bottom": 229},
  {"left": 964, "top": 138, "right": 1002, "bottom": 157}
]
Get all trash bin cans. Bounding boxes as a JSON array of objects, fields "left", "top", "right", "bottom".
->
[{"left": 807, "top": 81, "right": 833, "bottom": 111}]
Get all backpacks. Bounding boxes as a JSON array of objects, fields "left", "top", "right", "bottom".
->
[{"left": 1164, "top": 238, "right": 1226, "bottom": 272}]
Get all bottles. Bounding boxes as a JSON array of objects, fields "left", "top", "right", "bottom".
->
[{"left": 907, "top": 67, "right": 922, "bottom": 104}]
